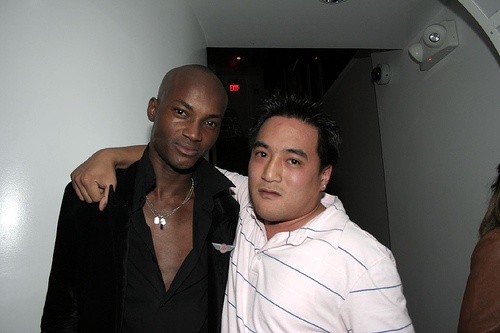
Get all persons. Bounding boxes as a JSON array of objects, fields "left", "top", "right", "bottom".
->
[
  {"left": 457, "top": 163, "right": 500, "bottom": 333},
  {"left": 40, "top": 66, "right": 239, "bottom": 333},
  {"left": 68, "top": 95, "right": 417, "bottom": 333}
]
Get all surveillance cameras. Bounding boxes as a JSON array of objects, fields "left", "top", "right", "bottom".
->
[{"left": 371, "top": 62, "right": 391, "bottom": 86}]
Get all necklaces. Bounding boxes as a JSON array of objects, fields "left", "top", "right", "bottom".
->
[{"left": 145, "top": 178, "right": 195, "bottom": 230}]
